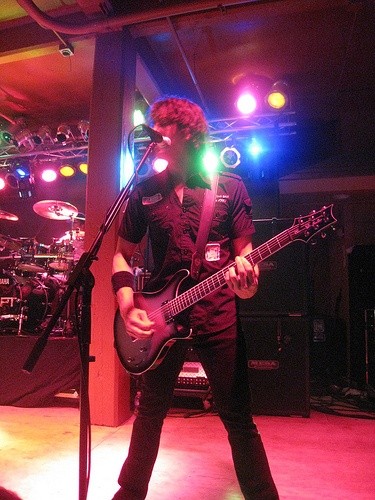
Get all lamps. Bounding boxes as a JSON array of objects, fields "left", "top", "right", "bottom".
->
[
  {"left": 33, "top": 124, "right": 51, "bottom": 146},
  {"left": 0, "top": 117, "right": 26, "bottom": 144},
  {"left": 57, "top": 122, "right": 71, "bottom": 141},
  {"left": 13, "top": 128, "right": 33, "bottom": 150},
  {"left": 264, "top": 79, "right": 291, "bottom": 111},
  {"left": 77, "top": 120, "right": 90, "bottom": 141}
]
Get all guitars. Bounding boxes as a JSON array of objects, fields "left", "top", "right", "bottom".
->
[{"left": 113, "top": 204, "right": 338, "bottom": 375}]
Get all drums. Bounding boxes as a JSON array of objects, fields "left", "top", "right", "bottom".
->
[
  {"left": 0, "top": 268, "right": 47, "bottom": 333},
  {"left": 15, "top": 261, "right": 48, "bottom": 298},
  {"left": 49, "top": 262, "right": 71, "bottom": 289}
]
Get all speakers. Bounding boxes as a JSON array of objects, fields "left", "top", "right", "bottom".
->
[
  {"left": 237, "top": 317, "right": 311, "bottom": 419},
  {"left": 234, "top": 218, "right": 314, "bottom": 318}
]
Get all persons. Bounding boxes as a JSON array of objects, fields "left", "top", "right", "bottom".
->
[
  {"left": 38, "top": 212, "right": 85, "bottom": 336},
  {"left": 111, "top": 95, "right": 279, "bottom": 500}
]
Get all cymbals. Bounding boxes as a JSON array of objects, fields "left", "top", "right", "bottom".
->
[
  {"left": 0, "top": 210, "right": 18, "bottom": 221},
  {"left": 34, "top": 200, "right": 78, "bottom": 220},
  {"left": 0, "top": 234, "right": 21, "bottom": 251}
]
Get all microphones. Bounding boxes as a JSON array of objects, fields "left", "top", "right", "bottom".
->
[{"left": 141, "top": 124, "right": 171, "bottom": 150}]
